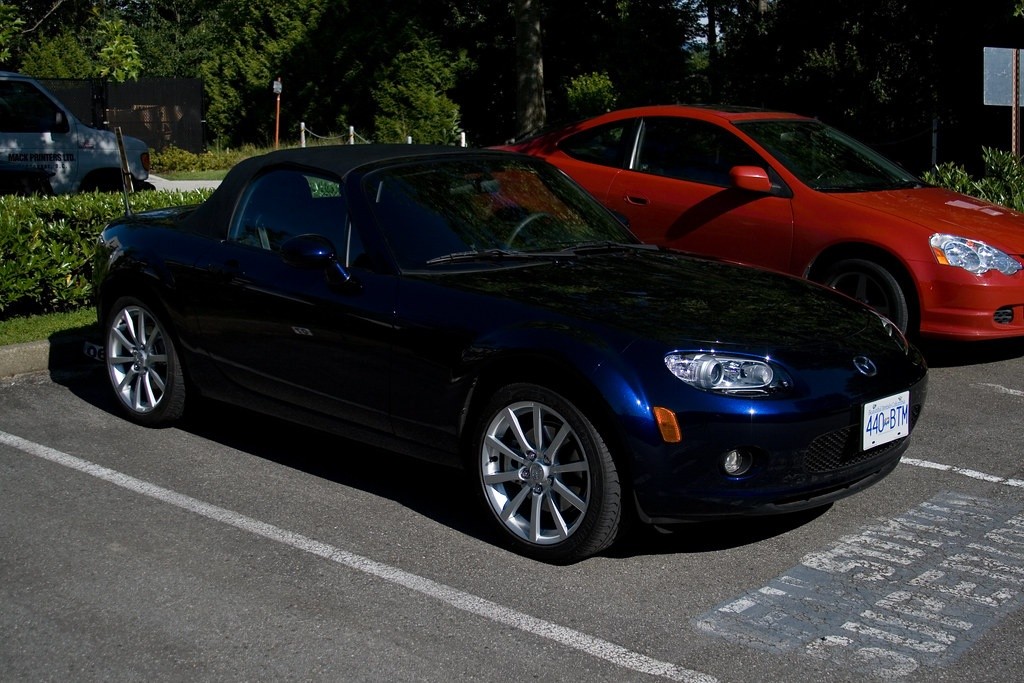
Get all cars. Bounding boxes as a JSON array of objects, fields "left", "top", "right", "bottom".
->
[
  {"left": 482, "top": 107, "right": 1024, "bottom": 356},
  {"left": 92, "top": 141, "right": 928, "bottom": 566}
]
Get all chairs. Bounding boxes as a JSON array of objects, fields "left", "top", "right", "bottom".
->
[{"left": 243, "top": 170, "right": 347, "bottom": 267}]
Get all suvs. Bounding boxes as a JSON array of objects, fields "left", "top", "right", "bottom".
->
[{"left": 0, "top": 72, "right": 149, "bottom": 198}]
